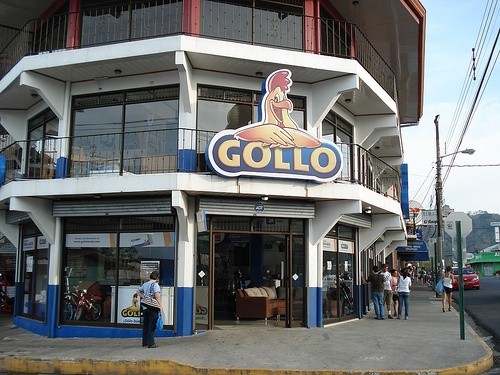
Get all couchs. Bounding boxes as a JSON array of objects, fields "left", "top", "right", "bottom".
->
[{"left": 234, "top": 289, "right": 343, "bottom": 325}]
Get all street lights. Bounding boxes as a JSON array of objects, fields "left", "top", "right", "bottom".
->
[{"left": 436, "top": 148, "right": 476, "bottom": 291}]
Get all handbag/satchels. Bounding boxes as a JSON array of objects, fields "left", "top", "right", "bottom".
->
[
  {"left": 140, "top": 283, "right": 160, "bottom": 310},
  {"left": 435, "top": 279, "right": 443, "bottom": 296}
]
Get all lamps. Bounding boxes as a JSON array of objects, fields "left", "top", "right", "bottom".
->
[
  {"left": 352, "top": 0, "right": 360, "bottom": 6},
  {"left": 345, "top": 99, "right": 351, "bottom": 104},
  {"left": 31, "top": 94, "right": 38, "bottom": 98},
  {"left": 256, "top": 72, "right": 263, "bottom": 76},
  {"left": 261, "top": 195, "right": 268, "bottom": 200},
  {"left": 365, "top": 207, "right": 371, "bottom": 213},
  {"left": 114, "top": 69, "right": 121, "bottom": 74}
]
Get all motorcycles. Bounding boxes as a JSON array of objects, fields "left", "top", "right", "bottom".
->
[{"left": 62, "top": 287, "right": 101, "bottom": 322}]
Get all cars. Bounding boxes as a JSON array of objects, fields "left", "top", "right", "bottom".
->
[{"left": 448, "top": 266, "right": 481, "bottom": 290}]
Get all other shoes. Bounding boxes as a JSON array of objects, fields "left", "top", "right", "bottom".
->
[
  {"left": 374, "top": 317, "right": 380, "bottom": 320},
  {"left": 381, "top": 317, "right": 384, "bottom": 320},
  {"left": 405, "top": 317, "right": 408, "bottom": 320},
  {"left": 443, "top": 308, "right": 445, "bottom": 312},
  {"left": 148, "top": 344, "right": 158, "bottom": 348},
  {"left": 394, "top": 312, "right": 397, "bottom": 317},
  {"left": 448, "top": 308, "right": 451, "bottom": 311},
  {"left": 388, "top": 314, "right": 393, "bottom": 319},
  {"left": 142, "top": 344, "right": 148, "bottom": 346}
]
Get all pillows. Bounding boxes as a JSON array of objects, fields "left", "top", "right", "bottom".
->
[{"left": 243, "top": 285, "right": 304, "bottom": 299}]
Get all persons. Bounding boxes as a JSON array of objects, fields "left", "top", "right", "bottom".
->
[
  {"left": 380, "top": 264, "right": 393, "bottom": 319},
  {"left": 138, "top": 271, "right": 163, "bottom": 348},
  {"left": 407, "top": 266, "right": 426, "bottom": 285},
  {"left": 441, "top": 267, "right": 455, "bottom": 312},
  {"left": 391, "top": 270, "right": 398, "bottom": 317},
  {"left": 362, "top": 266, "right": 386, "bottom": 319},
  {"left": 397, "top": 268, "right": 411, "bottom": 320}
]
